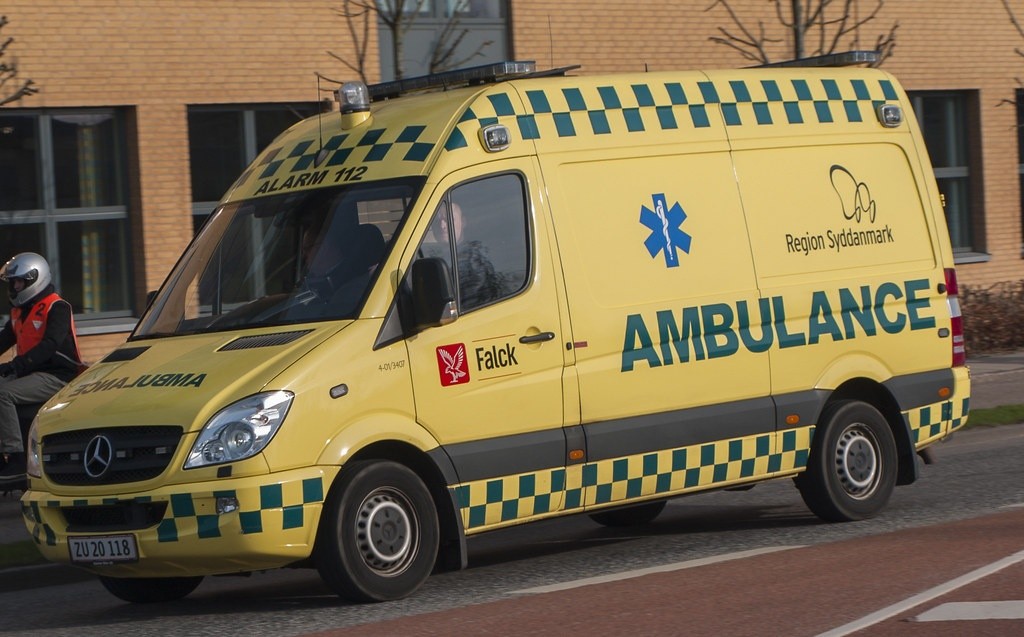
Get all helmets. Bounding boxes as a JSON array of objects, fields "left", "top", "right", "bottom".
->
[{"left": 0, "top": 252, "right": 51, "bottom": 306}]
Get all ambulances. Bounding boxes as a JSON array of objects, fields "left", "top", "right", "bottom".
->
[{"left": 21, "top": 51, "right": 972, "bottom": 604}]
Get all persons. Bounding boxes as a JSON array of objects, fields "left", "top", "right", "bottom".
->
[
  {"left": 419, "top": 200, "right": 496, "bottom": 316},
  {"left": 282, "top": 221, "right": 355, "bottom": 302},
  {"left": 0, "top": 252, "right": 84, "bottom": 479}
]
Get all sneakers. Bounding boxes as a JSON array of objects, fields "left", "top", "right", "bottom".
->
[{"left": 0, "top": 452, "right": 27, "bottom": 480}]
